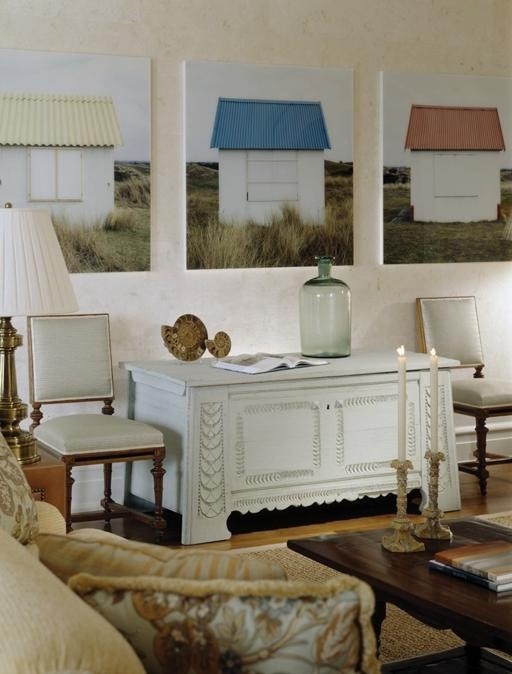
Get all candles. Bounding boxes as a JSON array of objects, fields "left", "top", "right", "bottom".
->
[
  {"left": 428, "top": 346, "right": 441, "bottom": 451},
  {"left": 395, "top": 344, "right": 409, "bottom": 462}
]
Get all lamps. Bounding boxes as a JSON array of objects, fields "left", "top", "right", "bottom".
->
[{"left": 1, "top": 198, "right": 81, "bottom": 467}]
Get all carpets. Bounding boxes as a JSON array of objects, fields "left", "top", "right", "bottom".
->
[{"left": 224, "top": 507, "right": 512, "bottom": 663}]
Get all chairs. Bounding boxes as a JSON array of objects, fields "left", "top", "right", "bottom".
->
[
  {"left": 415, "top": 293, "right": 511, "bottom": 495},
  {"left": 26, "top": 310, "right": 169, "bottom": 546}
]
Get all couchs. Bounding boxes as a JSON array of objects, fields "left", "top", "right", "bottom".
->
[{"left": 0, "top": 491, "right": 386, "bottom": 674}]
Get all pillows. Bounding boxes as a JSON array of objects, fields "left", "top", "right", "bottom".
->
[
  {"left": 64, "top": 566, "right": 385, "bottom": 674},
  {"left": 0, "top": 432, "right": 39, "bottom": 544},
  {"left": 35, "top": 523, "right": 289, "bottom": 589}
]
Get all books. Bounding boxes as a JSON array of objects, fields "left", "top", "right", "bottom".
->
[
  {"left": 428, "top": 559, "right": 512, "bottom": 593},
  {"left": 210, "top": 351, "right": 328, "bottom": 375},
  {"left": 434, "top": 538, "right": 512, "bottom": 584}
]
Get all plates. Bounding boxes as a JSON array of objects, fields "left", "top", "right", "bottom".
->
[
  {"left": 160, "top": 314, "right": 208, "bottom": 363},
  {"left": 203, "top": 329, "right": 232, "bottom": 358}
]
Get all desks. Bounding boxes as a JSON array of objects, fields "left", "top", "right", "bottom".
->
[
  {"left": 16, "top": 447, "right": 70, "bottom": 530},
  {"left": 119, "top": 344, "right": 463, "bottom": 549}
]
